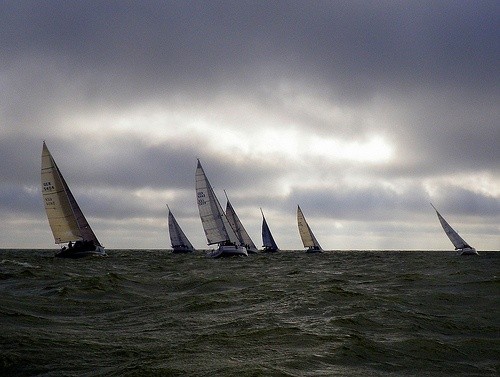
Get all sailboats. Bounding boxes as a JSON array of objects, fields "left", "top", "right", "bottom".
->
[
  {"left": 166, "top": 204, "right": 195, "bottom": 253},
  {"left": 260, "top": 208, "right": 279, "bottom": 254},
  {"left": 223, "top": 190, "right": 258, "bottom": 254},
  {"left": 430, "top": 202, "right": 477, "bottom": 256},
  {"left": 41, "top": 140, "right": 107, "bottom": 258},
  {"left": 297, "top": 205, "right": 323, "bottom": 254},
  {"left": 195, "top": 158, "right": 248, "bottom": 260}
]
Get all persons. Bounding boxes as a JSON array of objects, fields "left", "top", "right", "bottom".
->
[
  {"left": 61, "top": 246, "right": 66, "bottom": 251},
  {"left": 68, "top": 241, "right": 75, "bottom": 248}
]
